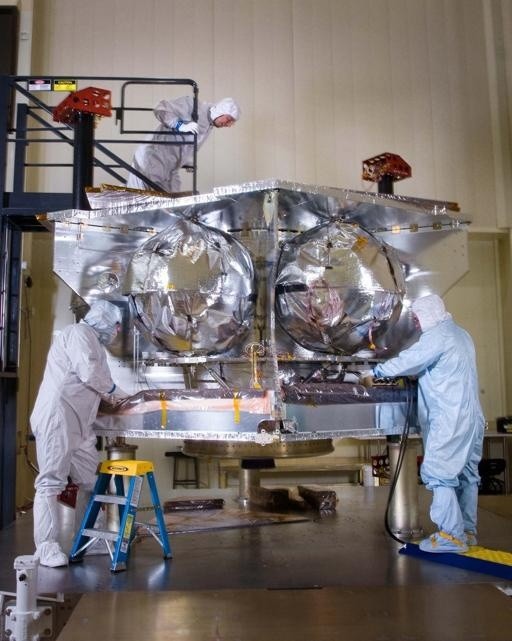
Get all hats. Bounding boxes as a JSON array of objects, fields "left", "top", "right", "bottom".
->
[
  {"left": 412, "top": 295, "right": 451, "bottom": 332},
  {"left": 84, "top": 299, "right": 122, "bottom": 344},
  {"left": 210, "top": 97, "right": 240, "bottom": 121}
]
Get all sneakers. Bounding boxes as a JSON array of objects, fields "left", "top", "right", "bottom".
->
[
  {"left": 419, "top": 530, "right": 477, "bottom": 553},
  {"left": 34, "top": 542, "right": 69, "bottom": 567}
]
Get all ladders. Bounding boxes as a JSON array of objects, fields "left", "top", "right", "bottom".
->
[{"left": 68, "top": 459, "right": 172, "bottom": 573}]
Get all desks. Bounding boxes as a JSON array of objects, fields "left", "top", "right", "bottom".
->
[
  {"left": 358, "top": 431, "right": 511, "bottom": 495},
  {"left": 165, "top": 451, "right": 200, "bottom": 489}
]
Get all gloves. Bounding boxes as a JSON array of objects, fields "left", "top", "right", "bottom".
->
[
  {"left": 176, "top": 121, "right": 201, "bottom": 135},
  {"left": 360, "top": 368, "right": 375, "bottom": 379},
  {"left": 100, "top": 385, "right": 129, "bottom": 405}
]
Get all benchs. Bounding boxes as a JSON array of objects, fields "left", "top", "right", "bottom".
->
[{"left": 217, "top": 458, "right": 370, "bottom": 488}]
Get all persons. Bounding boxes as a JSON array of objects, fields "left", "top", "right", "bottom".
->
[
  {"left": 361, "top": 294, "right": 487, "bottom": 554},
  {"left": 126, "top": 97, "right": 240, "bottom": 192},
  {"left": 29, "top": 300, "right": 134, "bottom": 567}
]
